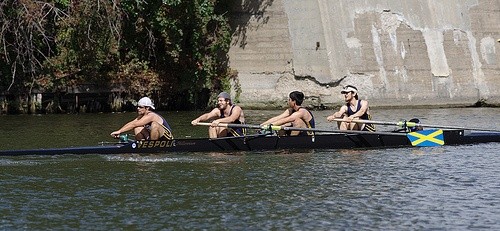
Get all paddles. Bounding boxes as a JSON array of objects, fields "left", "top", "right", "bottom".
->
[
  {"left": 193, "top": 121, "right": 444, "bottom": 148},
  {"left": 331, "top": 117, "right": 500, "bottom": 132}
]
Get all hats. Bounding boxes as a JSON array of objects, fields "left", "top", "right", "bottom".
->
[
  {"left": 341, "top": 86, "right": 357, "bottom": 94},
  {"left": 218, "top": 92, "right": 230, "bottom": 100},
  {"left": 137, "top": 97, "right": 155, "bottom": 109}
]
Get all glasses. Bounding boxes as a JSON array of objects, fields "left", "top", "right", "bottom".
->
[{"left": 136, "top": 105, "right": 145, "bottom": 109}]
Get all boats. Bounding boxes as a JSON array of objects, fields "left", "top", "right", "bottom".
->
[{"left": 0, "top": 127, "right": 500, "bottom": 156}]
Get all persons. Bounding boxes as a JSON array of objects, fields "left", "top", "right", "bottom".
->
[
  {"left": 111, "top": 97, "right": 173, "bottom": 140},
  {"left": 260, "top": 91, "right": 315, "bottom": 136},
  {"left": 191, "top": 92, "right": 246, "bottom": 138},
  {"left": 327, "top": 85, "right": 376, "bottom": 133}
]
